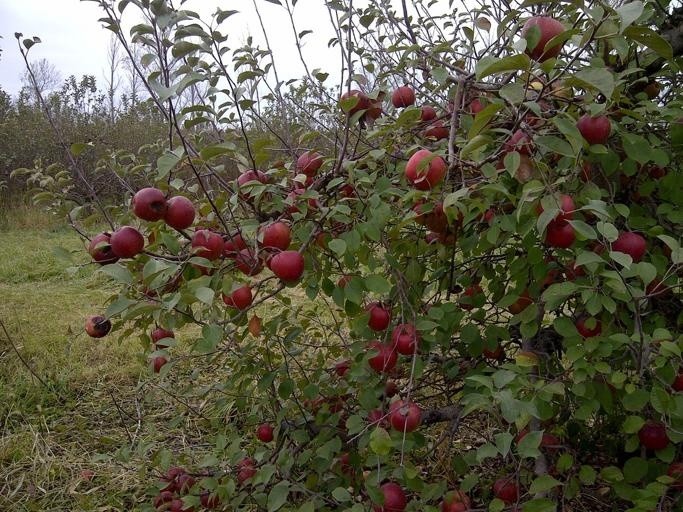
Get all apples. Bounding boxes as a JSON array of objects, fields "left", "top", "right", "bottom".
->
[
  {"left": 521, "top": 17, "right": 564, "bottom": 62},
  {"left": 84, "top": 83, "right": 683, "bottom": 512}
]
